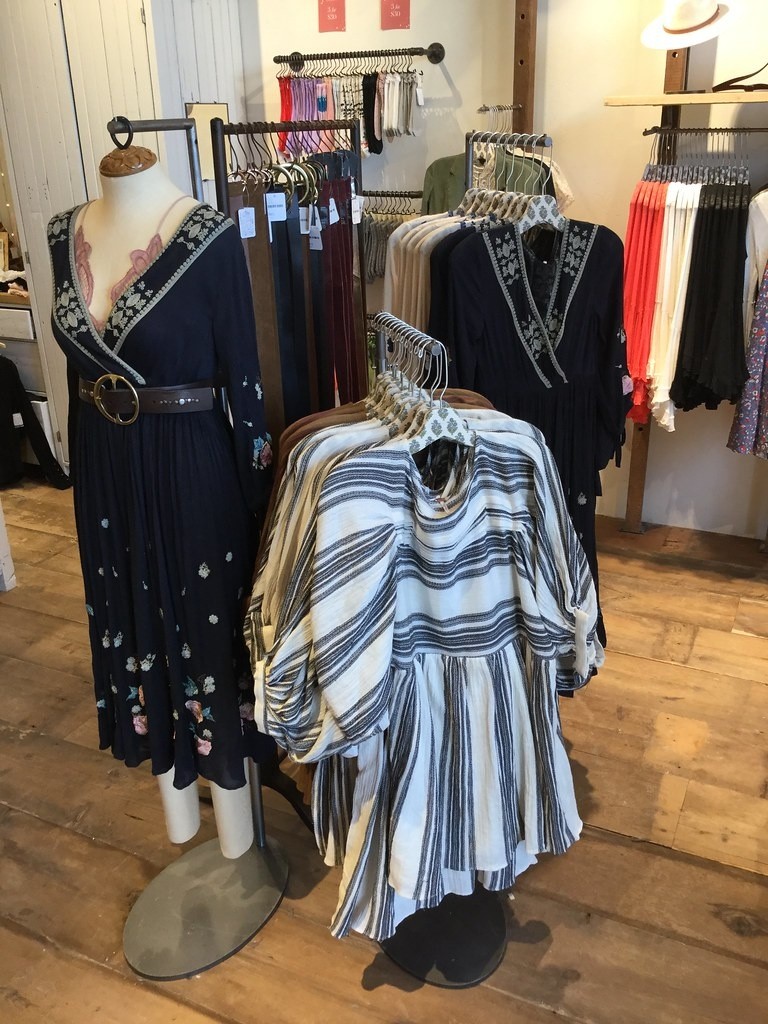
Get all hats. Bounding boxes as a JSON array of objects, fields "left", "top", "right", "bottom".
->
[{"left": 641, "top": 0, "right": 739, "bottom": 50}]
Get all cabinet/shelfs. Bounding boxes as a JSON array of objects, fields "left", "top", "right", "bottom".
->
[
  {"left": 0, "top": 1, "right": 167, "bottom": 469},
  {"left": 211, "top": 45, "right": 768, "bottom": 467}
]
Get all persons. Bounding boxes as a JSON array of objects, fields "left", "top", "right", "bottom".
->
[{"left": 44, "top": 149, "right": 278, "bottom": 859}]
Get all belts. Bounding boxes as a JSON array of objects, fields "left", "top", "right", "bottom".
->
[
  {"left": 228, "top": 144, "right": 373, "bottom": 476},
  {"left": 75, "top": 374, "right": 216, "bottom": 426}
]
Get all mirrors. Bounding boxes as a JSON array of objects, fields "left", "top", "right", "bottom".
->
[
  {"left": 149, "top": 0, "right": 258, "bottom": 204},
  {"left": 186, "top": 102, "right": 234, "bottom": 180}
]
